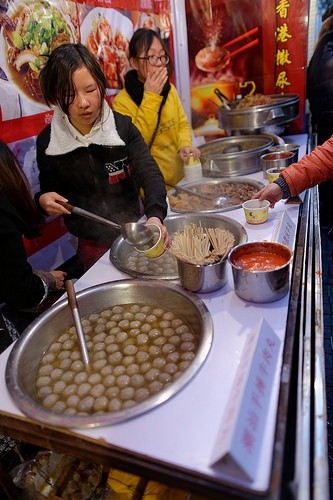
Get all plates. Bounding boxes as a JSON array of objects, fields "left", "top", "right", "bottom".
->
[{"left": 79, "top": 7, "right": 134, "bottom": 96}]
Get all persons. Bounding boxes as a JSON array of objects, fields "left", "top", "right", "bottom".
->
[
  {"left": 306, "top": 5, "right": 333, "bottom": 236},
  {"left": 250, "top": 132, "right": 333, "bottom": 210},
  {"left": 110, "top": 29, "right": 202, "bottom": 204},
  {"left": 0, "top": 140, "right": 68, "bottom": 354},
  {"left": 21, "top": 43, "right": 169, "bottom": 277}
]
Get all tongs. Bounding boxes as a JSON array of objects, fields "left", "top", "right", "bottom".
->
[{"left": 214, "top": 89, "right": 235, "bottom": 112}]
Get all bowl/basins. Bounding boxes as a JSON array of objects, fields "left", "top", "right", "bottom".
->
[
  {"left": 242, "top": 199, "right": 270, "bottom": 225},
  {"left": 260, "top": 151, "right": 296, "bottom": 179},
  {"left": 269, "top": 142, "right": 300, "bottom": 164},
  {"left": 110, "top": 213, "right": 248, "bottom": 280},
  {"left": 169, "top": 178, "right": 264, "bottom": 213},
  {"left": 227, "top": 238, "right": 292, "bottom": 303},
  {"left": 0, "top": 0, "right": 77, "bottom": 112},
  {"left": 266, "top": 167, "right": 283, "bottom": 184},
  {"left": 134, "top": 224, "right": 165, "bottom": 260},
  {"left": 171, "top": 255, "right": 230, "bottom": 292},
  {"left": 5, "top": 277, "right": 216, "bottom": 429}
]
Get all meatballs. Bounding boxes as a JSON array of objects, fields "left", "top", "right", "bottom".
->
[{"left": 34, "top": 230, "right": 197, "bottom": 418}]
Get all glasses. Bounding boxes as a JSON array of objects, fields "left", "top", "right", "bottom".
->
[{"left": 136, "top": 54, "right": 169, "bottom": 67}]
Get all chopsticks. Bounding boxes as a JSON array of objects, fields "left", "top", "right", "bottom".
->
[{"left": 218, "top": 26, "right": 259, "bottom": 63}]
